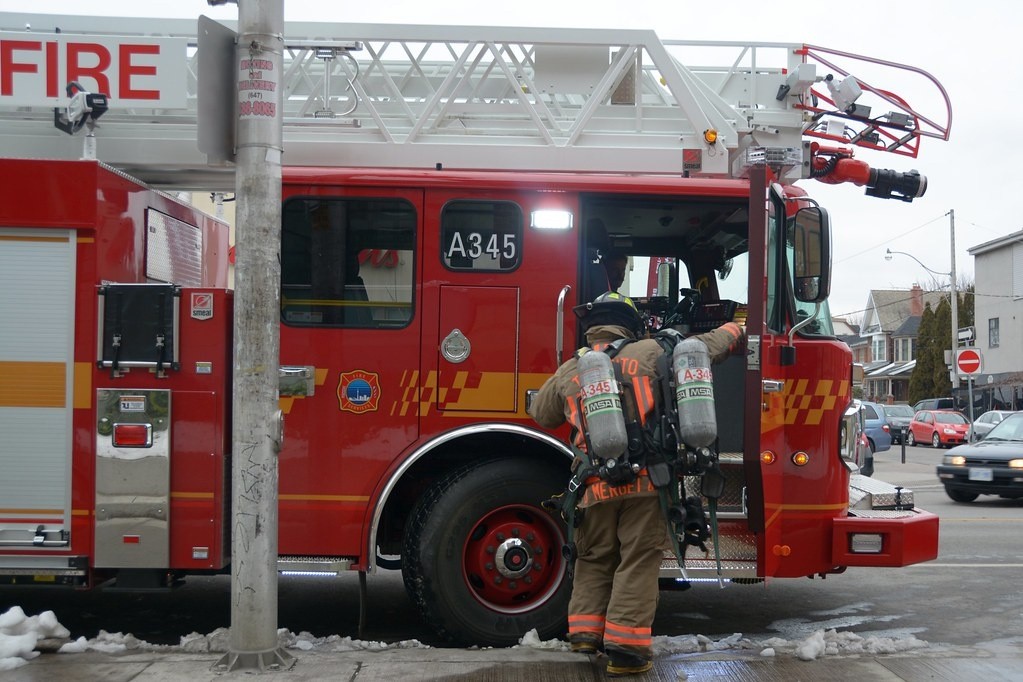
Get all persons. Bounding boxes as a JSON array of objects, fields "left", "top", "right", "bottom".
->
[
  {"left": 601, "top": 250, "right": 627, "bottom": 293},
  {"left": 525, "top": 292, "right": 745, "bottom": 674}
]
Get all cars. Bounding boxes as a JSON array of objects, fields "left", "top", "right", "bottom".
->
[
  {"left": 935, "top": 411, "right": 1023, "bottom": 504},
  {"left": 966, "top": 410, "right": 1017, "bottom": 443},
  {"left": 908, "top": 410, "right": 968, "bottom": 447},
  {"left": 880, "top": 404, "right": 915, "bottom": 443},
  {"left": 857, "top": 398, "right": 891, "bottom": 454}
]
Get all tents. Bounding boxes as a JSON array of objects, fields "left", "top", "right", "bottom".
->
[{"left": 974, "top": 372, "right": 1023, "bottom": 412}]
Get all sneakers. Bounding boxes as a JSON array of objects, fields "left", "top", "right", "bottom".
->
[
  {"left": 569, "top": 639, "right": 598, "bottom": 652},
  {"left": 606, "top": 652, "right": 652, "bottom": 675}
]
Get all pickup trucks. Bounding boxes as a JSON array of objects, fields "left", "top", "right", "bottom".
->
[{"left": 912, "top": 397, "right": 964, "bottom": 412}]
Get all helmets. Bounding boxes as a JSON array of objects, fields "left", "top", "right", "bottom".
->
[{"left": 575, "top": 288, "right": 642, "bottom": 334}]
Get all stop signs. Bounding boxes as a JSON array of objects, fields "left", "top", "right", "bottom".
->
[{"left": 955, "top": 348, "right": 982, "bottom": 376}]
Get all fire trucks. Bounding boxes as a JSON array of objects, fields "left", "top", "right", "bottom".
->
[{"left": 1, "top": 8, "right": 952, "bottom": 646}]
